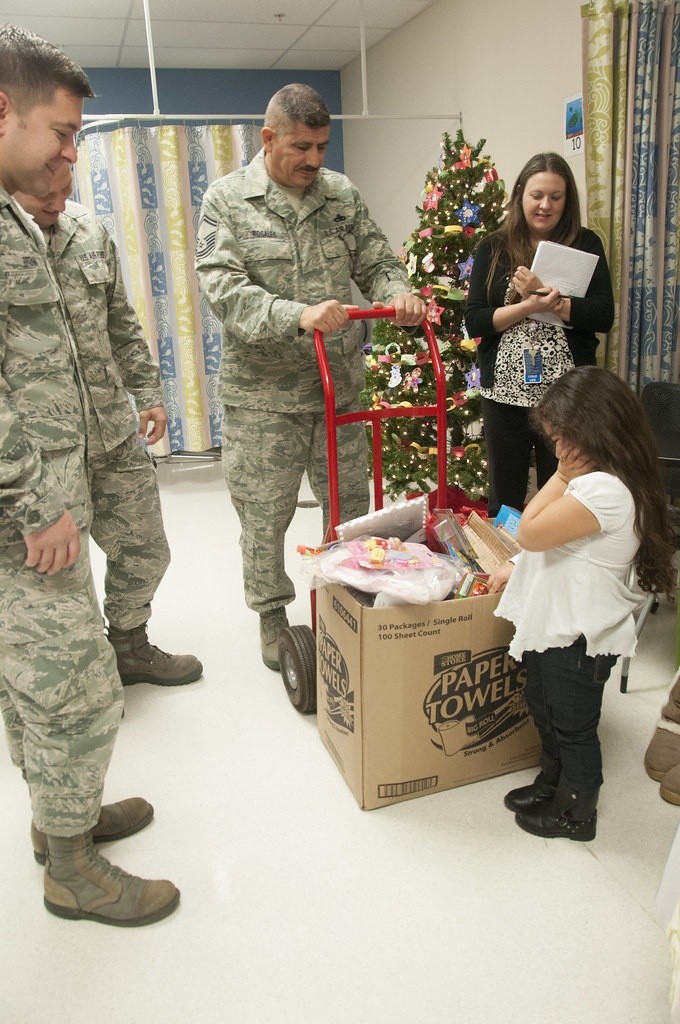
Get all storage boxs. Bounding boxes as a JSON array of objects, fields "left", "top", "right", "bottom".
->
[{"left": 315, "top": 564, "right": 543, "bottom": 812}]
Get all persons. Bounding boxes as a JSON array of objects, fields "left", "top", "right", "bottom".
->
[
  {"left": 464, "top": 151, "right": 616, "bottom": 517},
  {"left": 0, "top": 23, "right": 181, "bottom": 927},
  {"left": 486, "top": 362, "right": 677, "bottom": 842},
  {"left": 12, "top": 163, "right": 202, "bottom": 687},
  {"left": 193, "top": 82, "right": 428, "bottom": 670}
]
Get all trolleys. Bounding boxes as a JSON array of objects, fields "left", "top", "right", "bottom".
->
[{"left": 275, "top": 310, "right": 451, "bottom": 711}]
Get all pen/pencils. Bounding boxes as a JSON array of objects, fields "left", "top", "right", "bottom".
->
[{"left": 528, "top": 290, "right": 573, "bottom": 299}]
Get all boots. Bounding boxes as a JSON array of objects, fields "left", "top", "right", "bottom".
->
[
  {"left": 39, "top": 830, "right": 181, "bottom": 929},
  {"left": 28, "top": 796, "right": 155, "bottom": 867},
  {"left": 504, "top": 753, "right": 563, "bottom": 813},
  {"left": 258, "top": 605, "right": 292, "bottom": 672},
  {"left": 519, "top": 769, "right": 600, "bottom": 844},
  {"left": 638, "top": 666, "right": 680, "bottom": 780},
  {"left": 658, "top": 762, "right": 680, "bottom": 806},
  {"left": 105, "top": 620, "right": 205, "bottom": 689}
]
